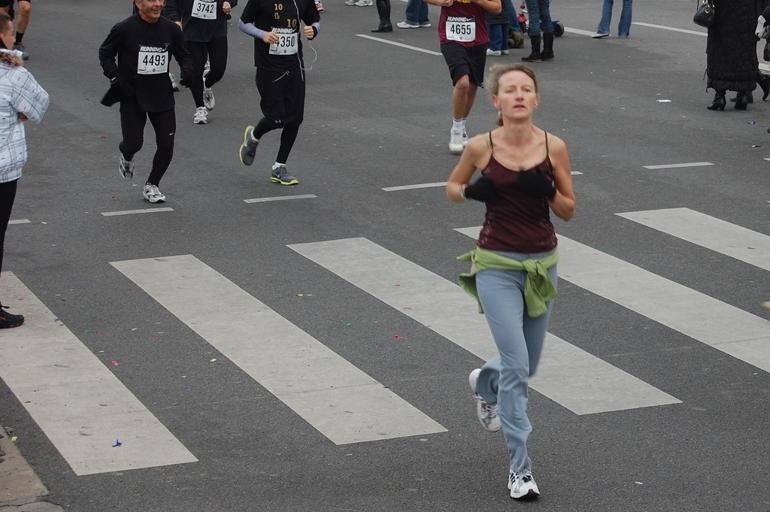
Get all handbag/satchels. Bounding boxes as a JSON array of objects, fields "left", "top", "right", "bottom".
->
[{"left": 693, "top": 0, "right": 716, "bottom": 29}]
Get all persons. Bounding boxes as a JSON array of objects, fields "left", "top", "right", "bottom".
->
[
  {"left": 237, "top": 1, "right": 320, "bottom": 185},
  {"left": 314, "top": 0, "right": 325, "bottom": 11},
  {"left": 427, "top": 1, "right": 503, "bottom": 153},
  {"left": 133, "top": 1, "right": 240, "bottom": 124},
  {"left": 345, "top": 1, "right": 433, "bottom": 34},
  {"left": 705, "top": 1, "right": 770, "bottom": 111},
  {"left": 0, "top": 1, "right": 33, "bottom": 62},
  {"left": 0, "top": 13, "right": 50, "bottom": 329},
  {"left": 446, "top": 66, "right": 577, "bottom": 497},
  {"left": 480, "top": 2, "right": 563, "bottom": 62},
  {"left": 590, "top": 1, "right": 636, "bottom": 39},
  {"left": 98, "top": 1, "right": 198, "bottom": 203}
]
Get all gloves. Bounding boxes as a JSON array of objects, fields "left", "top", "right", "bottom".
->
[
  {"left": 516, "top": 166, "right": 557, "bottom": 201},
  {"left": 464, "top": 177, "right": 503, "bottom": 203}
]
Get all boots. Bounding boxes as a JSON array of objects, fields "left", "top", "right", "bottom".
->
[
  {"left": 540, "top": 32, "right": 555, "bottom": 61},
  {"left": 521, "top": 34, "right": 541, "bottom": 62}
]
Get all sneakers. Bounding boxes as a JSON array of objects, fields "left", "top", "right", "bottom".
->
[
  {"left": 142, "top": 180, "right": 167, "bottom": 204},
  {"left": 201, "top": 77, "right": 216, "bottom": 111},
  {"left": 370, "top": 18, "right": 432, "bottom": 32},
  {"left": 118, "top": 151, "right": 136, "bottom": 182},
  {"left": 591, "top": 31, "right": 609, "bottom": 38},
  {"left": 192, "top": 105, "right": 209, "bottom": 125},
  {"left": 467, "top": 367, "right": 504, "bottom": 433},
  {"left": 345, "top": 0, "right": 374, "bottom": 7},
  {"left": 12, "top": 41, "right": 30, "bottom": 60},
  {"left": 168, "top": 74, "right": 179, "bottom": 92},
  {"left": 315, "top": 1, "right": 326, "bottom": 12},
  {"left": 448, "top": 126, "right": 469, "bottom": 155},
  {"left": 0, "top": 303, "right": 26, "bottom": 329},
  {"left": 506, "top": 466, "right": 541, "bottom": 500},
  {"left": 270, "top": 164, "right": 300, "bottom": 187},
  {"left": 237, "top": 124, "right": 259, "bottom": 168},
  {"left": 485, "top": 47, "right": 510, "bottom": 57}
]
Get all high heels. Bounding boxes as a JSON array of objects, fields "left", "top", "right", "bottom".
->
[
  {"left": 734, "top": 95, "right": 748, "bottom": 111},
  {"left": 707, "top": 96, "right": 727, "bottom": 112}
]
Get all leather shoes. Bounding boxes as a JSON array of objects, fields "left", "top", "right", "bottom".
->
[
  {"left": 762, "top": 75, "right": 770, "bottom": 101},
  {"left": 730, "top": 93, "right": 753, "bottom": 104}
]
